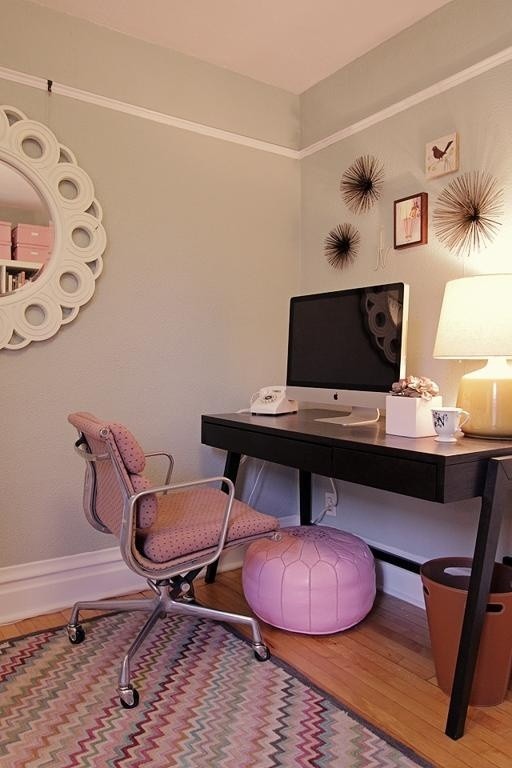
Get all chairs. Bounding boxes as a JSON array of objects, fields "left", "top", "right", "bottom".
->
[{"left": 65, "top": 410, "right": 283, "bottom": 709}]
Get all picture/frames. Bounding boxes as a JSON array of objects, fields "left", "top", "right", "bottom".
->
[{"left": 393, "top": 191, "right": 428, "bottom": 250}]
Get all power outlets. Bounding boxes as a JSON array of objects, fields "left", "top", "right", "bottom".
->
[{"left": 324, "top": 491, "right": 338, "bottom": 518}]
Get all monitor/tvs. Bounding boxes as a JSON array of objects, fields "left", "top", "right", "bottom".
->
[{"left": 284, "top": 280, "right": 410, "bottom": 429}]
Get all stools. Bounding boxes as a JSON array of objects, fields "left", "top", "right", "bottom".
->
[{"left": 241, "top": 525, "right": 378, "bottom": 636}]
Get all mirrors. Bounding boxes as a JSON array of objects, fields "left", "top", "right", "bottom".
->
[{"left": 0, "top": 103, "right": 108, "bottom": 352}]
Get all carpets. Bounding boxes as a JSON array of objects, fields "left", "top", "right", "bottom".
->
[{"left": 0, "top": 593, "right": 436, "bottom": 768}]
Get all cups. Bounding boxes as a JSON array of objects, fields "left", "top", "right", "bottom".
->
[{"left": 431, "top": 406, "right": 471, "bottom": 443}]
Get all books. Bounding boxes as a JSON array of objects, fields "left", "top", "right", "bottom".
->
[{"left": 0, "top": 263, "right": 32, "bottom": 295}]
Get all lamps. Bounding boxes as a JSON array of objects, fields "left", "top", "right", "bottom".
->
[{"left": 432, "top": 272, "right": 512, "bottom": 442}]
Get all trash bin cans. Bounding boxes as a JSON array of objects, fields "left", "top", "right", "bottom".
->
[{"left": 421, "top": 556, "right": 512, "bottom": 706}]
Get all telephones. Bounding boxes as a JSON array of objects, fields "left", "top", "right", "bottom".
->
[{"left": 250, "top": 385, "right": 298, "bottom": 416}]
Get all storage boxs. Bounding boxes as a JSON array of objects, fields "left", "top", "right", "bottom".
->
[{"left": 1, "top": 221, "right": 52, "bottom": 262}]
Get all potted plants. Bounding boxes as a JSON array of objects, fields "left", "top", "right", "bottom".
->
[{"left": 384, "top": 375, "right": 443, "bottom": 440}]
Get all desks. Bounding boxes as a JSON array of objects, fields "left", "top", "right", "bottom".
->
[{"left": 200, "top": 407, "right": 512, "bottom": 742}]
